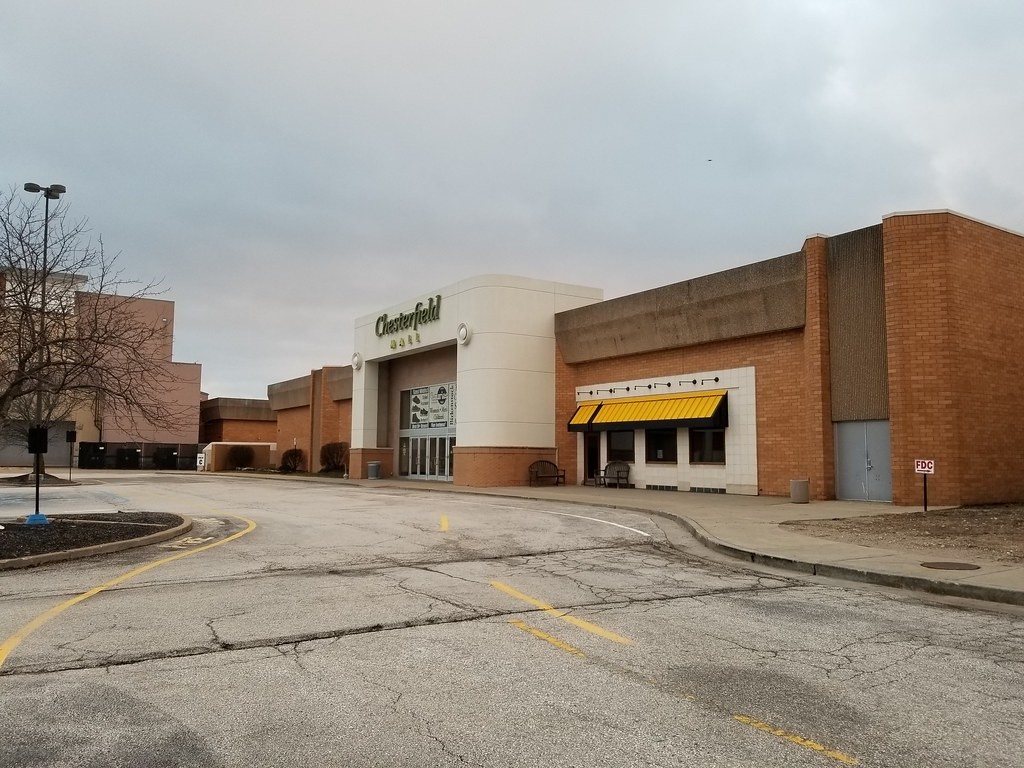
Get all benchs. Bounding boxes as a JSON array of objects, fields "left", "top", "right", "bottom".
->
[
  {"left": 528, "top": 460, "right": 566, "bottom": 488},
  {"left": 594, "top": 461, "right": 631, "bottom": 490}
]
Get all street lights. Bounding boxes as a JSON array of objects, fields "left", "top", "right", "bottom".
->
[{"left": 22, "top": 179, "right": 66, "bottom": 529}]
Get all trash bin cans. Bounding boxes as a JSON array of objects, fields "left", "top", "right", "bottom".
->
[
  {"left": 789, "top": 480, "right": 809, "bottom": 504},
  {"left": 84, "top": 444, "right": 107, "bottom": 469},
  {"left": 153, "top": 447, "right": 177, "bottom": 471},
  {"left": 115, "top": 447, "right": 141, "bottom": 470},
  {"left": 365, "top": 460, "right": 383, "bottom": 479}
]
